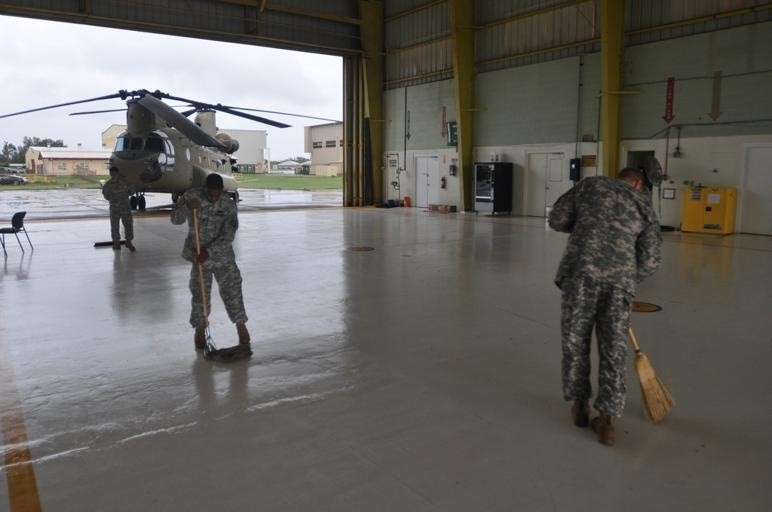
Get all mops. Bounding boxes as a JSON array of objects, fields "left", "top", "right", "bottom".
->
[
  {"left": 93, "top": 238, "right": 126, "bottom": 246},
  {"left": 190, "top": 207, "right": 253, "bottom": 362}
]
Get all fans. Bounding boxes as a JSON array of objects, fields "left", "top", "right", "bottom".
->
[{"left": 644, "top": 155, "right": 675, "bottom": 232}]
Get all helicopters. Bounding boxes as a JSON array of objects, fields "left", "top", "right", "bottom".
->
[{"left": 0, "top": 89, "right": 343, "bottom": 212}]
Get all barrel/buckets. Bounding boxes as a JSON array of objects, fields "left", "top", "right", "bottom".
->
[
  {"left": 404, "top": 196, "right": 410, "bottom": 207},
  {"left": 388, "top": 199, "right": 393, "bottom": 206}
]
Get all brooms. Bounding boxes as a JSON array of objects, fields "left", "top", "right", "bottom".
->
[{"left": 629, "top": 326, "right": 676, "bottom": 426}]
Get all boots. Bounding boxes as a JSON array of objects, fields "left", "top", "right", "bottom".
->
[
  {"left": 571, "top": 398, "right": 589, "bottom": 428},
  {"left": 589, "top": 411, "right": 615, "bottom": 446},
  {"left": 113, "top": 239, "right": 135, "bottom": 252},
  {"left": 195, "top": 326, "right": 206, "bottom": 348},
  {"left": 237, "top": 323, "right": 250, "bottom": 344}
]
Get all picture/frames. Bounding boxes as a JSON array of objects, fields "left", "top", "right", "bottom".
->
[{"left": 663, "top": 188, "right": 675, "bottom": 199}]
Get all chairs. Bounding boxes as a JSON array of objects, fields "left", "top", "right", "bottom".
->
[{"left": 0, "top": 211, "right": 33, "bottom": 256}]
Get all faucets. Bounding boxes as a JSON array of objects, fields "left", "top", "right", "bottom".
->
[{"left": 392, "top": 182, "right": 399, "bottom": 190}]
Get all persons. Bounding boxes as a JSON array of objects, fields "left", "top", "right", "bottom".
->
[
  {"left": 171, "top": 174, "right": 250, "bottom": 348},
  {"left": 548, "top": 167, "right": 663, "bottom": 447},
  {"left": 102, "top": 167, "right": 137, "bottom": 252}
]
